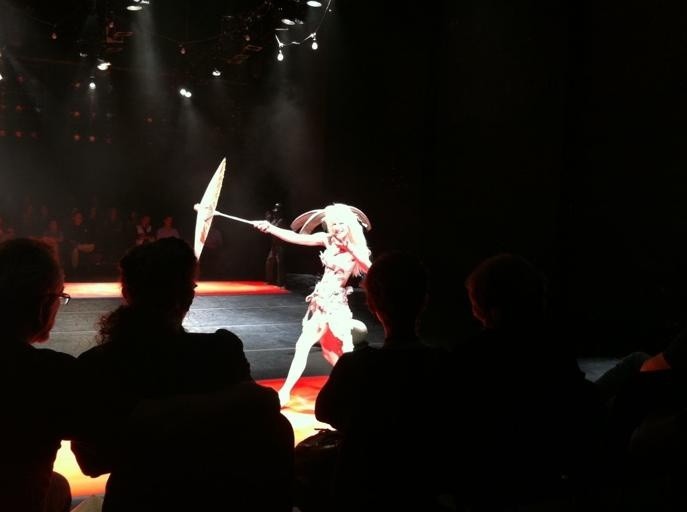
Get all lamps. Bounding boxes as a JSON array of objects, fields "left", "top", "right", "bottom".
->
[{"left": 271, "top": 1, "right": 325, "bottom": 49}]
